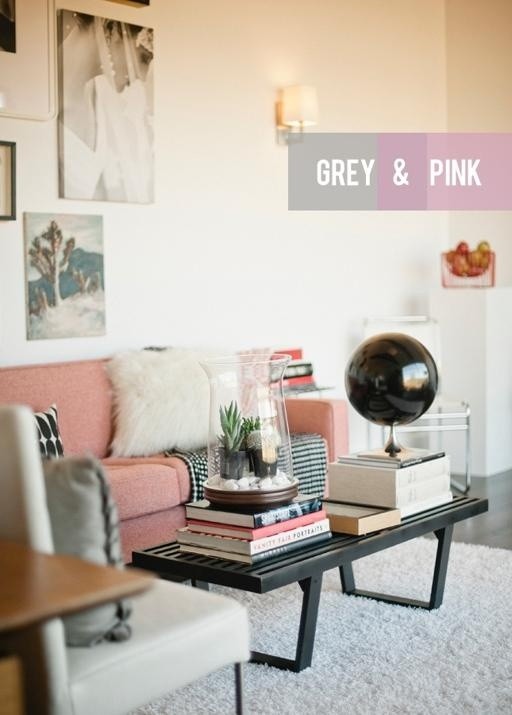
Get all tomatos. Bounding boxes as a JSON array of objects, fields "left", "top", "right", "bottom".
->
[{"left": 447, "top": 243, "right": 468, "bottom": 273}]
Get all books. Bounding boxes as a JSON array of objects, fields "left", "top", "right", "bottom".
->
[
  {"left": 235, "top": 347, "right": 314, "bottom": 392},
  {"left": 172, "top": 495, "right": 335, "bottom": 566},
  {"left": 338, "top": 449, "right": 446, "bottom": 469}
]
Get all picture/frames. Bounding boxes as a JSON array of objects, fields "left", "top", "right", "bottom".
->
[{"left": 0, "top": 0, "right": 55, "bottom": 221}]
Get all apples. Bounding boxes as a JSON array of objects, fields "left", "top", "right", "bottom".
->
[{"left": 478, "top": 242, "right": 489, "bottom": 251}]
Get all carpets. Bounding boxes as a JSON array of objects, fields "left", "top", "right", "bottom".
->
[{"left": 129, "top": 536, "right": 510, "bottom": 714}]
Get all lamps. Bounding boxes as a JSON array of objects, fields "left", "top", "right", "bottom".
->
[{"left": 278, "top": 84, "right": 320, "bottom": 134}]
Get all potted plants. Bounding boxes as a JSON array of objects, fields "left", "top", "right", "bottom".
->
[{"left": 211, "top": 400, "right": 279, "bottom": 479}]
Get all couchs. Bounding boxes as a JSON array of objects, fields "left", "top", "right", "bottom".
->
[{"left": 0, "top": 344, "right": 348, "bottom": 594}]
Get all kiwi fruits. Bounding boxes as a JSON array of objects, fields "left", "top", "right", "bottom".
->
[{"left": 467, "top": 251, "right": 488, "bottom": 267}]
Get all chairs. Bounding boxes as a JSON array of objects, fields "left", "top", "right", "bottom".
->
[{"left": 0, "top": 404, "right": 255, "bottom": 715}]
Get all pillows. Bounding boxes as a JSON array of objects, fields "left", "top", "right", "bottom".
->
[
  {"left": 108, "top": 346, "right": 248, "bottom": 457},
  {"left": 38, "top": 402, "right": 136, "bottom": 639}
]
